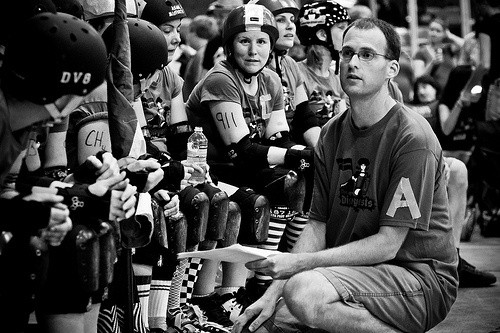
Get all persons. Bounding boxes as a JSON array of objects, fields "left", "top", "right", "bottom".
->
[{"left": 0, "top": 0, "right": 500, "bottom": 333}]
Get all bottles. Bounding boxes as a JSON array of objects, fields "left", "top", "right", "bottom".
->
[{"left": 187, "top": 127, "right": 207, "bottom": 184}]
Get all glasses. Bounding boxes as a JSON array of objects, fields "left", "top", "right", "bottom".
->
[{"left": 338, "top": 49, "right": 394, "bottom": 63}]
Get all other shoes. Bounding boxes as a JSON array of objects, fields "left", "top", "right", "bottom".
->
[{"left": 457, "top": 254, "right": 497, "bottom": 288}]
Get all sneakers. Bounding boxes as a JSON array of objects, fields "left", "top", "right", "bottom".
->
[{"left": 149, "top": 278, "right": 274, "bottom": 333}]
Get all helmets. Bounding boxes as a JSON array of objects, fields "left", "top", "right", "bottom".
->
[
  {"left": 0, "top": 0, "right": 108, "bottom": 105},
  {"left": 223, "top": 4, "right": 281, "bottom": 44},
  {"left": 102, "top": 18, "right": 168, "bottom": 79},
  {"left": 256, "top": 0, "right": 301, "bottom": 18},
  {"left": 297, "top": 2, "right": 352, "bottom": 46},
  {"left": 140, "top": 0, "right": 188, "bottom": 26}
]
